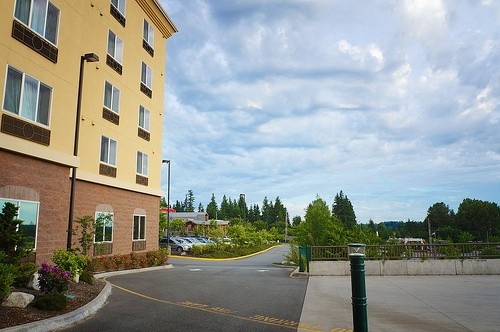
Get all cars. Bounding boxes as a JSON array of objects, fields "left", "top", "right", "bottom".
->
[{"left": 161, "top": 236, "right": 218, "bottom": 256}]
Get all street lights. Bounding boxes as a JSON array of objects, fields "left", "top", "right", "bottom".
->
[
  {"left": 240, "top": 194, "right": 246, "bottom": 227},
  {"left": 486, "top": 228, "right": 491, "bottom": 247},
  {"left": 162, "top": 159, "right": 170, "bottom": 255},
  {"left": 66, "top": 52, "right": 100, "bottom": 253}
]
